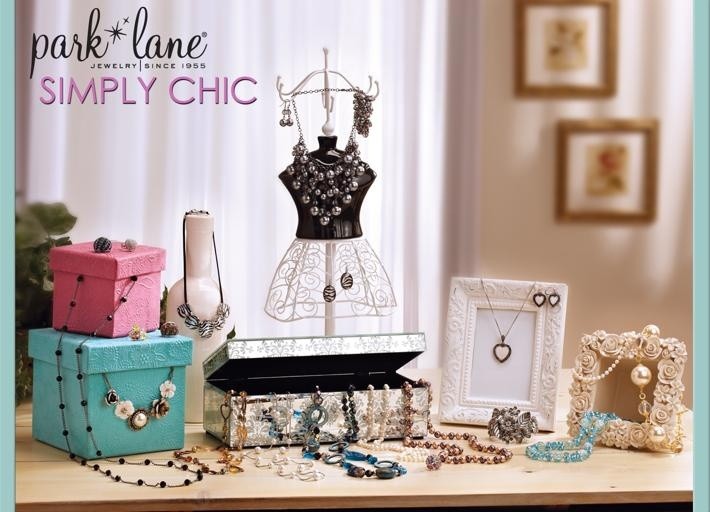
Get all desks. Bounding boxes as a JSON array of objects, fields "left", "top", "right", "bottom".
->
[{"left": 15, "top": 368, "right": 694, "bottom": 512}]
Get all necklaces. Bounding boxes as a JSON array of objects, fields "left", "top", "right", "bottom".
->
[
  {"left": 243, "top": 394, "right": 324, "bottom": 481},
  {"left": 526, "top": 410, "right": 619, "bottom": 461},
  {"left": 301, "top": 386, "right": 407, "bottom": 479},
  {"left": 288, "top": 89, "right": 365, "bottom": 226},
  {"left": 358, "top": 383, "right": 430, "bottom": 464},
  {"left": 55, "top": 276, "right": 202, "bottom": 489},
  {"left": 479, "top": 276, "right": 535, "bottom": 364},
  {"left": 172, "top": 390, "right": 248, "bottom": 480},
  {"left": 100, "top": 366, "right": 178, "bottom": 430},
  {"left": 572, "top": 330, "right": 626, "bottom": 382},
  {"left": 633, "top": 325, "right": 683, "bottom": 454},
  {"left": 177, "top": 209, "right": 230, "bottom": 340},
  {"left": 401, "top": 377, "right": 512, "bottom": 470}
]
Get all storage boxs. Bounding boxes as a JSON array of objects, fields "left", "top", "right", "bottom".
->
[
  {"left": 202, "top": 333, "right": 431, "bottom": 448},
  {"left": 51, "top": 239, "right": 166, "bottom": 339},
  {"left": 27, "top": 327, "right": 193, "bottom": 459}
]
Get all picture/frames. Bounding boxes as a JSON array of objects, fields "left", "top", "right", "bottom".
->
[
  {"left": 567, "top": 323, "right": 690, "bottom": 454},
  {"left": 437, "top": 276, "right": 569, "bottom": 433},
  {"left": 554, "top": 116, "right": 661, "bottom": 226},
  {"left": 513, "top": 0, "right": 620, "bottom": 99}
]
